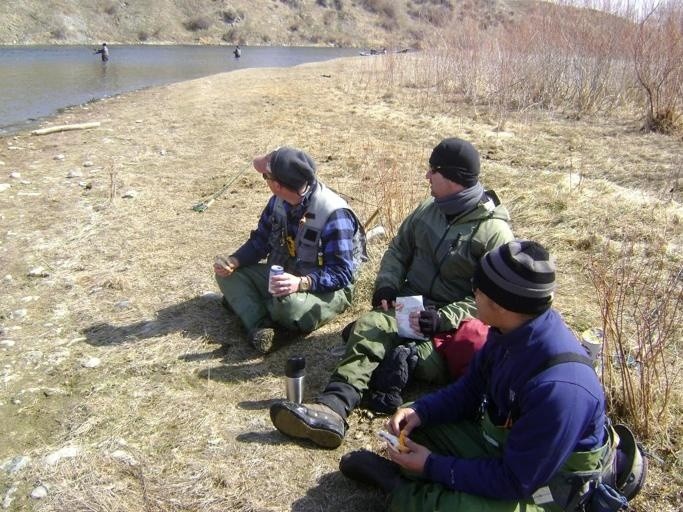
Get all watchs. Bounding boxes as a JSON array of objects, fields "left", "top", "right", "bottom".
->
[{"left": 300, "top": 277, "right": 310, "bottom": 292}]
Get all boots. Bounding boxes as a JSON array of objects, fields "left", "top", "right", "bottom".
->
[{"left": 339, "top": 449, "right": 413, "bottom": 505}]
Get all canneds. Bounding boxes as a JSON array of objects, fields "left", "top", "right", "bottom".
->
[{"left": 268, "top": 265, "right": 284, "bottom": 293}]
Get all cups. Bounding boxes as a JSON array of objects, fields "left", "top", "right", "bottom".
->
[{"left": 582, "top": 329, "right": 603, "bottom": 361}]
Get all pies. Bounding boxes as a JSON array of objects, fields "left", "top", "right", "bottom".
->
[
  {"left": 214, "top": 254, "right": 235, "bottom": 273},
  {"left": 378, "top": 431, "right": 410, "bottom": 454}
]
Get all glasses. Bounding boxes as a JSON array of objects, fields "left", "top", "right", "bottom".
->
[
  {"left": 470, "top": 277, "right": 483, "bottom": 298},
  {"left": 430, "top": 164, "right": 472, "bottom": 174},
  {"left": 262, "top": 173, "right": 279, "bottom": 183}
]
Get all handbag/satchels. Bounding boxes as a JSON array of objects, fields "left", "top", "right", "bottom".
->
[
  {"left": 584, "top": 423, "right": 648, "bottom": 511},
  {"left": 434, "top": 318, "right": 489, "bottom": 380}
]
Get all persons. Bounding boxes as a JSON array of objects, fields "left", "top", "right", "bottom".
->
[
  {"left": 370, "top": 47, "right": 387, "bottom": 54},
  {"left": 233, "top": 45, "right": 241, "bottom": 59},
  {"left": 270, "top": 139, "right": 514, "bottom": 450},
  {"left": 339, "top": 239, "right": 605, "bottom": 510},
  {"left": 213, "top": 147, "right": 362, "bottom": 354},
  {"left": 94, "top": 43, "right": 109, "bottom": 63}
]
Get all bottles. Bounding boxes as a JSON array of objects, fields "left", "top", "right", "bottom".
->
[{"left": 285, "top": 355, "right": 306, "bottom": 405}]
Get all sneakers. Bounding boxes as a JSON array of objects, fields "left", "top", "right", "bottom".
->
[
  {"left": 341, "top": 321, "right": 357, "bottom": 342},
  {"left": 248, "top": 318, "right": 291, "bottom": 354},
  {"left": 221, "top": 296, "right": 236, "bottom": 312},
  {"left": 270, "top": 401, "right": 345, "bottom": 448}
]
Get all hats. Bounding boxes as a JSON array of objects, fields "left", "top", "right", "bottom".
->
[
  {"left": 476, "top": 240, "right": 556, "bottom": 314},
  {"left": 253, "top": 147, "right": 316, "bottom": 188},
  {"left": 429, "top": 138, "right": 480, "bottom": 187}
]
